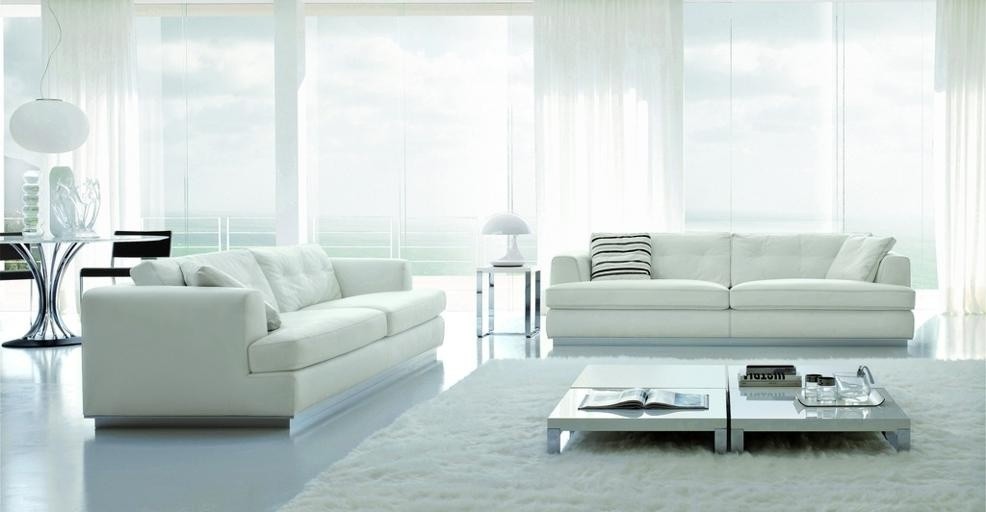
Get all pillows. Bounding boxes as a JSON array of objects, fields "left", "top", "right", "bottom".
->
[
  {"left": 821, "top": 235, "right": 899, "bottom": 284},
  {"left": 585, "top": 229, "right": 654, "bottom": 282},
  {"left": 186, "top": 265, "right": 282, "bottom": 333},
  {"left": 172, "top": 246, "right": 280, "bottom": 316},
  {"left": 246, "top": 239, "right": 346, "bottom": 312}
]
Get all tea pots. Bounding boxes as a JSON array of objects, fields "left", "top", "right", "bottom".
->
[{"left": 833, "top": 365, "right": 875, "bottom": 401}]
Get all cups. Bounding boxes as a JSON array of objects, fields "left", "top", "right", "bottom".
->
[
  {"left": 805, "top": 374, "right": 823, "bottom": 401},
  {"left": 816, "top": 376, "right": 837, "bottom": 401}
]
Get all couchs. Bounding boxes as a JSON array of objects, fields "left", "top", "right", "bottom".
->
[
  {"left": 80, "top": 245, "right": 450, "bottom": 437},
  {"left": 537, "top": 231, "right": 918, "bottom": 353}
]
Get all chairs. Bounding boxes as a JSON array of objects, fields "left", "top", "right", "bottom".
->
[
  {"left": 0, "top": 229, "right": 50, "bottom": 329},
  {"left": 72, "top": 226, "right": 172, "bottom": 319}
]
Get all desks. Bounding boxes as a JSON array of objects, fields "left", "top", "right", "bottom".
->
[
  {"left": 0, "top": 230, "right": 172, "bottom": 352},
  {"left": 469, "top": 260, "right": 542, "bottom": 339}
]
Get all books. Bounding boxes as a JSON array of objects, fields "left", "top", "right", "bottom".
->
[
  {"left": 737, "top": 364, "right": 802, "bottom": 387},
  {"left": 577, "top": 387, "right": 709, "bottom": 410}
]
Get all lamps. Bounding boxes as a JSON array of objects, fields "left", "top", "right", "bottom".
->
[
  {"left": 6, "top": 0, "right": 91, "bottom": 157},
  {"left": 481, "top": 210, "right": 533, "bottom": 270}
]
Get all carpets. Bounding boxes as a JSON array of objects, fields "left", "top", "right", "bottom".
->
[{"left": 270, "top": 347, "right": 986, "bottom": 512}]
